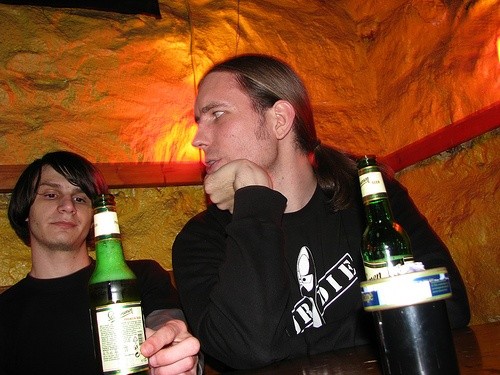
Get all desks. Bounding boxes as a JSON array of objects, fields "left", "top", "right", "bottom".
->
[{"left": 247, "top": 320, "right": 500, "bottom": 375}]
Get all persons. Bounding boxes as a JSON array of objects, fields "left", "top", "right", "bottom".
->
[
  {"left": 0, "top": 151, "right": 203, "bottom": 375},
  {"left": 170, "top": 54, "right": 471, "bottom": 369}
]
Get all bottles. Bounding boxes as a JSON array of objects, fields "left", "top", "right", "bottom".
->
[
  {"left": 85, "top": 194, "right": 153, "bottom": 375},
  {"left": 354, "top": 155, "right": 415, "bottom": 281}
]
